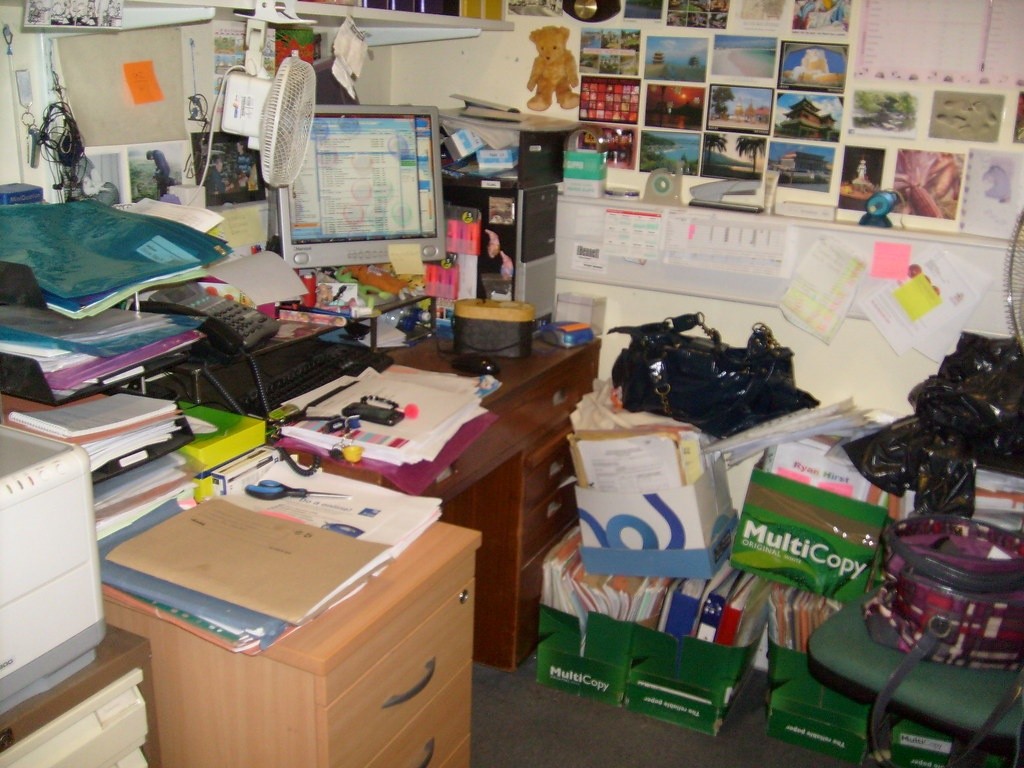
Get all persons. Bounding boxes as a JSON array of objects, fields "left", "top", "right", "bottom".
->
[
  {"left": 235, "top": 141, "right": 256, "bottom": 192},
  {"left": 208, "top": 154, "right": 226, "bottom": 196},
  {"left": 145, "top": 150, "right": 171, "bottom": 198}
]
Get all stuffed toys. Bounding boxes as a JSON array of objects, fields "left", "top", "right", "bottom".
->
[{"left": 526, "top": 25, "right": 581, "bottom": 112}]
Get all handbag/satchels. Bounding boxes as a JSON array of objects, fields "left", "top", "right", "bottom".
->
[
  {"left": 861, "top": 513, "right": 1024, "bottom": 768},
  {"left": 607, "top": 312, "right": 820, "bottom": 441}
]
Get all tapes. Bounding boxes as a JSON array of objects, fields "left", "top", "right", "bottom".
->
[
  {"left": 652, "top": 172, "right": 674, "bottom": 196},
  {"left": 564, "top": 124, "right": 607, "bottom": 155}
]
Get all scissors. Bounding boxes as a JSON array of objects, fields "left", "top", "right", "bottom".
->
[{"left": 244, "top": 479, "right": 352, "bottom": 502}]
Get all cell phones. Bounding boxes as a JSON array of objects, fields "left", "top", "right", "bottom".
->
[{"left": 343, "top": 401, "right": 405, "bottom": 426}]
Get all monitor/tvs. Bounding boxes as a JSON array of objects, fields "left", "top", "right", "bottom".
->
[{"left": 276, "top": 104, "right": 446, "bottom": 269}]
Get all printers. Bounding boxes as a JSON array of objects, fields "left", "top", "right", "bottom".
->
[{"left": 0, "top": 424, "right": 106, "bottom": 715}]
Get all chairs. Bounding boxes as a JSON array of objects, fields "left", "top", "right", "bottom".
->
[{"left": 807, "top": 582, "right": 1023, "bottom": 768}]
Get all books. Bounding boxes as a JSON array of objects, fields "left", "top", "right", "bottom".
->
[
  {"left": 540, "top": 528, "right": 845, "bottom": 652},
  {"left": 703, "top": 396, "right": 870, "bottom": 468},
  {"left": 566, "top": 420, "right": 712, "bottom": 493},
  {"left": 453, "top": 91, "right": 528, "bottom": 122},
  {"left": 0, "top": 200, "right": 212, "bottom": 399},
  {"left": 759, "top": 437, "right": 918, "bottom": 522},
  {"left": 3, "top": 394, "right": 182, "bottom": 475},
  {"left": 97, "top": 498, "right": 394, "bottom": 659},
  {"left": 290, "top": 363, "right": 503, "bottom": 453}
]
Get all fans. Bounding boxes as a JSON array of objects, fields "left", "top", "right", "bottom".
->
[{"left": 219, "top": 2, "right": 317, "bottom": 187}]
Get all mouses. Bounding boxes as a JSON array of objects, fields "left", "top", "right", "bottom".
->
[{"left": 450, "top": 349, "right": 501, "bottom": 376}]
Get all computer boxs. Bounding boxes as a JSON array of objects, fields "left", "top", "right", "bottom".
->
[{"left": 444, "top": 184, "right": 558, "bottom": 332}]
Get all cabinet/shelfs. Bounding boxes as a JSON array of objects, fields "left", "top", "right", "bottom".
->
[
  {"left": 103, "top": 520, "right": 484, "bottom": 767},
  {"left": 264, "top": 321, "right": 603, "bottom": 673}
]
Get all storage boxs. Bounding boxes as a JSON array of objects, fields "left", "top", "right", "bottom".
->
[
  {"left": 562, "top": 149, "right": 608, "bottom": 199},
  {"left": 535, "top": 310, "right": 1024, "bottom": 768},
  {"left": 774, "top": 200, "right": 839, "bottom": 222}
]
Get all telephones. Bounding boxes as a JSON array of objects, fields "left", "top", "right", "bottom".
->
[{"left": 122, "top": 280, "right": 282, "bottom": 366}]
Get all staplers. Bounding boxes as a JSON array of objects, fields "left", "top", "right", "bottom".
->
[{"left": 688, "top": 179, "right": 762, "bottom": 214}]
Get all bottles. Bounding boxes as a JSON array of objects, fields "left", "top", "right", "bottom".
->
[{"left": 391, "top": 306, "right": 433, "bottom": 332}]
